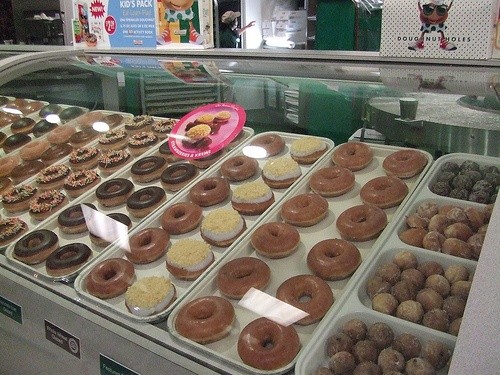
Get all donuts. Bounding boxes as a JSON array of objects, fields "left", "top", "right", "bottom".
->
[
  {"left": 0, "top": 96, "right": 176, "bottom": 245},
  {"left": 12, "top": 128, "right": 427, "bottom": 371},
  {"left": 85, "top": 34, "right": 97, "bottom": 43}
]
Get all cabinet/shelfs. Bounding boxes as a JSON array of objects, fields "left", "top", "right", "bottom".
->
[
  {"left": 281, "top": 0, "right": 356, "bottom": 136},
  {"left": 139, "top": 73, "right": 224, "bottom": 117}
]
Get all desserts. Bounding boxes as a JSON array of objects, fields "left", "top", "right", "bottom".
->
[{"left": 182, "top": 110, "right": 232, "bottom": 147}]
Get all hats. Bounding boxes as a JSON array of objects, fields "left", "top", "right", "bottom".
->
[{"left": 222, "top": 10, "right": 241, "bottom": 24}]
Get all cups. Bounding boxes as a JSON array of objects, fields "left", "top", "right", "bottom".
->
[{"left": 399, "top": 98, "right": 418, "bottom": 121}]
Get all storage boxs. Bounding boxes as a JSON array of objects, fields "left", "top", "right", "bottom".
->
[{"left": 27, "top": 18, "right": 60, "bottom": 38}]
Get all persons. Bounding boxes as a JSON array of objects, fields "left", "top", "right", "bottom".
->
[{"left": 218, "top": 11, "right": 256, "bottom": 103}]
